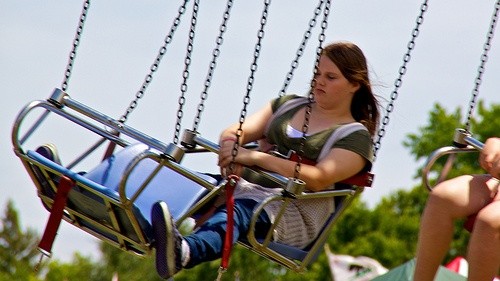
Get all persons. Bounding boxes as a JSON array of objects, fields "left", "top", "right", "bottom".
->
[
  {"left": 412, "top": 137, "right": 500, "bottom": 281},
  {"left": 33, "top": 42, "right": 389, "bottom": 280}
]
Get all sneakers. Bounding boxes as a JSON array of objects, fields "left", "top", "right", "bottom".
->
[
  {"left": 30, "top": 143, "right": 65, "bottom": 208},
  {"left": 150, "top": 199, "right": 183, "bottom": 276}
]
